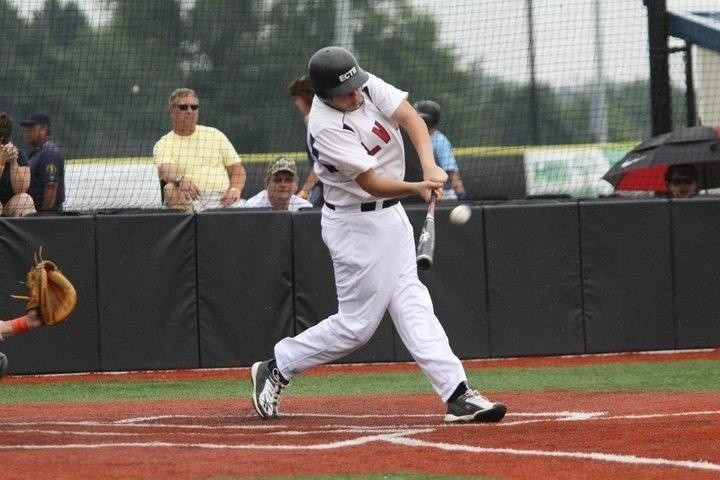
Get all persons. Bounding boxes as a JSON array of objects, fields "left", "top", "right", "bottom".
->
[
  {"left": 153, "top": 88, "right": 247, "bottom": 213},
  {"left": 0, "top": 260, "right": 78, "bottom": 376},
  {"left": 288, "top": 74, "right": 323, "bottom": 210},
  {"left": 411, "top": 100, "right": 468, "bottom": 202},
  {"left": 247, "top": 156, "right": 312, "bottom": 212},
  {"left": 251, "top": 46, "right": 506, "bottom": 425},
  {"left": 1, "top": 110, "right": 37, "bottom": 217},
  {"left": 20, "top": 113, "right": 65, "bottom": 212},
  {"left": 664, "top": 164, "right": 699, "bottom": 197}
]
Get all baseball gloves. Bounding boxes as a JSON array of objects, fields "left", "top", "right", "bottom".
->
[{"left": 11, "top": 246, "right": 76, "bottom": 325}]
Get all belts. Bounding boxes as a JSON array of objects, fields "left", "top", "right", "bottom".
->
[{"left": 324, "top": 195, "right": 397, "bottom": 212}]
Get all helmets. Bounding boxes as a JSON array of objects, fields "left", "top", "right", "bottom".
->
[{"left": 307, "top": 45, "right": 369, "bottom": 98}]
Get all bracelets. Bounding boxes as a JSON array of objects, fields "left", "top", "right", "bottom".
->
[
  {"left": 227, "top": 187, "right": 240, "bottom": 193},
  {"left": 457, "top": 192, "right": 467, "bottom": 201},
  {"left": 10, "top": 315, "right": 29, "bottom": 335}
]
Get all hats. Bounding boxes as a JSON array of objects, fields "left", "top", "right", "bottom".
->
[
  {"left": 414, "top": 101, "right": 440, "bottom": 125},
  {"left": 266, "top": 156, "right": 297, "bottom": 176},
  {"left": 20, "top": 114, "right": 51, "bottom": 126}
]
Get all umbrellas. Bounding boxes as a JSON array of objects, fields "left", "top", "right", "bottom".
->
[{"left": 602, "top": 114, "right": 719, "bottom": 194}]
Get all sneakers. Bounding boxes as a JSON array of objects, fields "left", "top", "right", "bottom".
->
[
  {"left": 443, "top": 389, "right": 507, "bottom": 423},
  {"left": 251, "top": 359, "right": 286, "bottom": 420}
]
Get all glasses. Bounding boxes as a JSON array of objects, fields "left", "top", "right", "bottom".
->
[
  {"left": 177, "top": 104, "right": 199, "bottom": 110},
  {"left": 668, "top": 176, "right": 692, "bottom": 185}
]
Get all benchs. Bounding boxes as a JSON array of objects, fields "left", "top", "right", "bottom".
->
[{"left": 160, "top": 154, "right": 525, "bottom": 205}]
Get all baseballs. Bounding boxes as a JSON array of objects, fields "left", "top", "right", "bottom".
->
[{"left": 450, "top": 205, "right": 471, "bottom": 225}]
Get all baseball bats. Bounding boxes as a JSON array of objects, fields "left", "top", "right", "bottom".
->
[{"left": 417, "top": 190, "right": 437, "bottom": 269}]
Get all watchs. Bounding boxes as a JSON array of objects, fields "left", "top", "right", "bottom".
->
[{"left": 176, "top": 176, "right": 186, "bottom": 187}]
